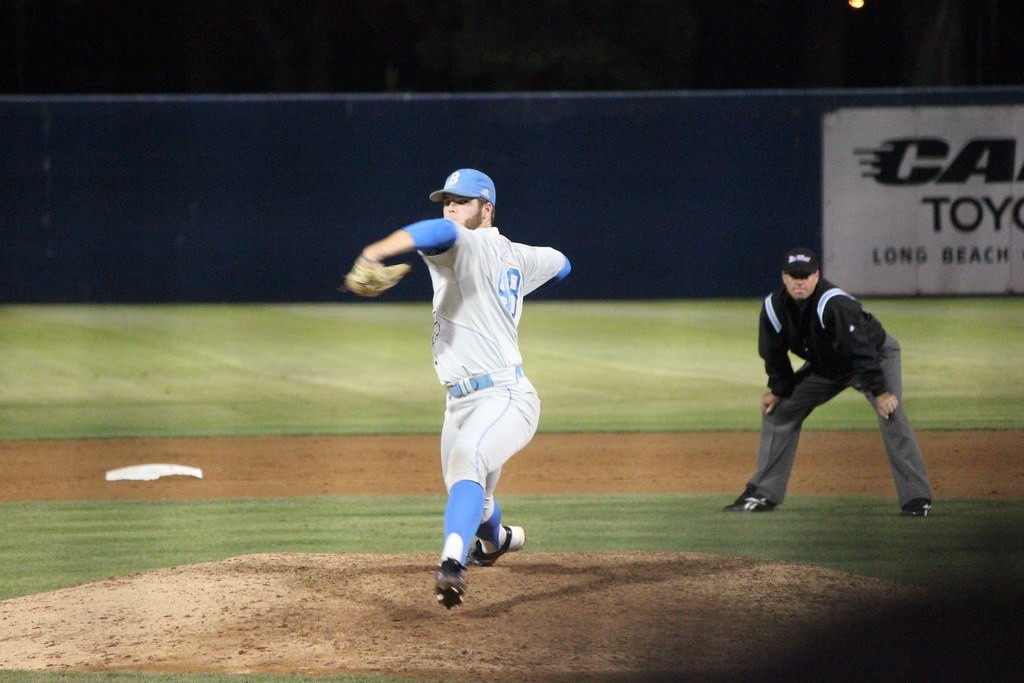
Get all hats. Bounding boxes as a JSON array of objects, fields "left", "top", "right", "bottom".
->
[
  {"left": 782, "top": 247, "right": 818, "bottom": 276},
  {"left": 429, "top": 169, "right": 496, "bottom": 206}
]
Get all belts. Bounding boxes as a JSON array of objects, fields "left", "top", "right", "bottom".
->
[{"left": 447, "top": 366, "right": 522, "bottom": 397}]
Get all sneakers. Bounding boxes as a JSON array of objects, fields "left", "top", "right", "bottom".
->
[
  {"left": 901, "top": 499, "right": 930, "bottom": 516},
  {"left": 435, "top": 556, "right": 462, "bottom": 609},
  {"left": 723, "top": 491, "right": 774, "bottom": 512},
  {"left": 472, "top": 525, "right": 525, "bottom": 566}
]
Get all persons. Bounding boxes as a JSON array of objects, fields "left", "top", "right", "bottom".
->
[
  {"left": 345, "top": 168, "right": 571, "bottom": 610},
  {"left": 723, "top": 248, "right": 933, "bottom": 518}
]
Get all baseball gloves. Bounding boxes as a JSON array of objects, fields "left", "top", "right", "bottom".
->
[{"left": 343, "top": 258, "right": 411, "bottom": 299}]
